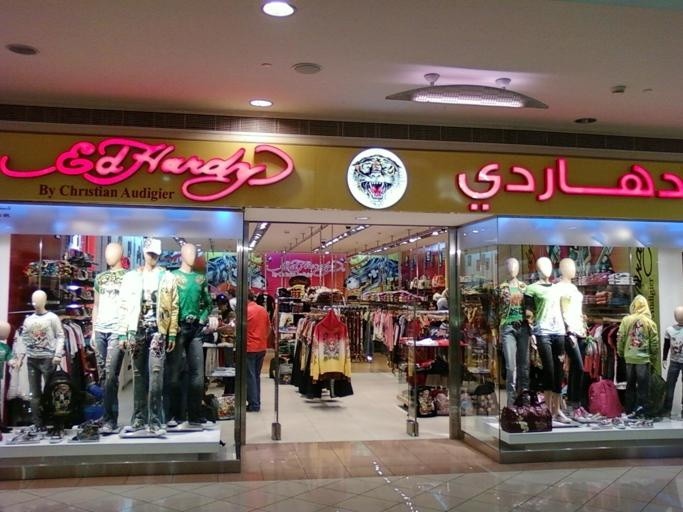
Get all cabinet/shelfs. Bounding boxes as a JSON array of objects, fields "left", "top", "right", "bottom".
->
[
  {"left": 398, "top": 338, "right": 491, "bottom": 414},
  {"left": 202, "top": 341, "right": 235, "bottom": 420},
  {"left": 577, "top": 284, "right": 635, "bottom": 316},
  {"left": 274, "top": 298, "right": 345, "bottom": 385},
  {"left": 22, "top": 258, "right": 100, "bottom": 404}
]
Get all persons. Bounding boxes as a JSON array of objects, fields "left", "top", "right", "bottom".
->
[
  {"left": 89, "top": 242, "right": 130, "bottom": 433},
  {"left": 524, "top": 257, "right": 578, "bottom": 424},
  {"left": 116, "top": 240, "right": 180, "bottom": 436},
  {"left": 215, "top": 287, "right": 295, "bottom": 413},
  {"left": 554, "top": 258, "right": 596, "bottom": 423},
  {"left": 0, "top": 321, "right": 17, "bottom": 434},
  {"left": 166, "top": 243, "right": 214, "bottom": 428},
  {"left": 202, "top": 393, "right": 221, "bottom": 420},
  {"left": 616, "top": 293, "right": 661, "bottom": 425},
  {"left": 487, "top": 257, "right": 532, "bottom": 416},
  {"left": 15, "top": 289, "right": 67, "bottom": 442},
  {"left": 662, "top": 307, "right": 683, "bottom": 420}
]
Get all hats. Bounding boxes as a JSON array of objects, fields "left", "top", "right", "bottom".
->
[{"left": 144, "top": 238, "right": 161, "bottom": 255}]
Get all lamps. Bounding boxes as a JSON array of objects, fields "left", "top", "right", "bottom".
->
[{"left": 385, "top": 73, "right": 549, "bottom": 109}]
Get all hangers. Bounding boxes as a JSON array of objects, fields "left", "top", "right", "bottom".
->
[
  {"left": 303, "top": 313, "right": 328, "bottom": 321},
  {"left": 370, "top": 301, "right": 414, "bottom": 310},
  {"left": 332, "top": 305, "right": 368, "bottom": 310},
  {"left": 408, "top": 310, "right": 449, "bottom": 319}
]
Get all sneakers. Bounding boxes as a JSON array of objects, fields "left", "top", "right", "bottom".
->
[
  {"left": 556, "top": 407, "right": 652, "bottom": 429},
  {"left": 24, "top": 418, "right": 213, "bottom": 441}
]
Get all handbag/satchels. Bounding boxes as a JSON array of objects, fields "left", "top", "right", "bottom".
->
[
  {"left": 501, "top": 391, "right": 553, "bottom": 433},
  {"left": 216, "top": 394, "right": 250, "bottom": 420},
  {"left": 644, "top": 372, "right": 668, "bottom": 417},
  {"left": 589, "top": 380, "right": 624, "bottom": 419},
  {"left": 43, "top": 370, "right": 76, "bottom": 419},
  {"left": 419, "top": 385, "right": 499, "bottom": 416}
]
